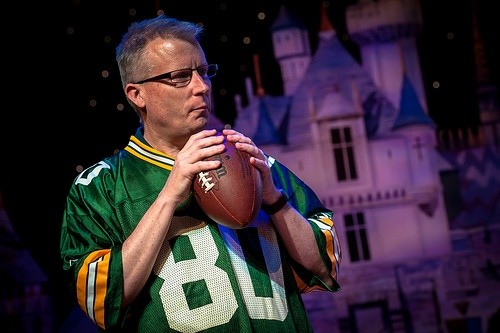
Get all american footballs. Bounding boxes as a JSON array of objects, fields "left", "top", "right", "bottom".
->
[{"left": 192, "top": 114, "right": 262, "bottom": 228}]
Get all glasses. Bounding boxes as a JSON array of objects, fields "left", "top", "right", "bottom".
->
[{"left": 134, "top": 63, "right": 218, "bottom": 84}]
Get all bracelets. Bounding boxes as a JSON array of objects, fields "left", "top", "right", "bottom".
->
[{"left": 262, "top": 189, "right": 290, "bottom": 216}]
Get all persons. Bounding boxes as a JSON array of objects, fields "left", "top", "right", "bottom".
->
[{"left": 60, "top": 14, "right": 342, "bottom": 333}]
